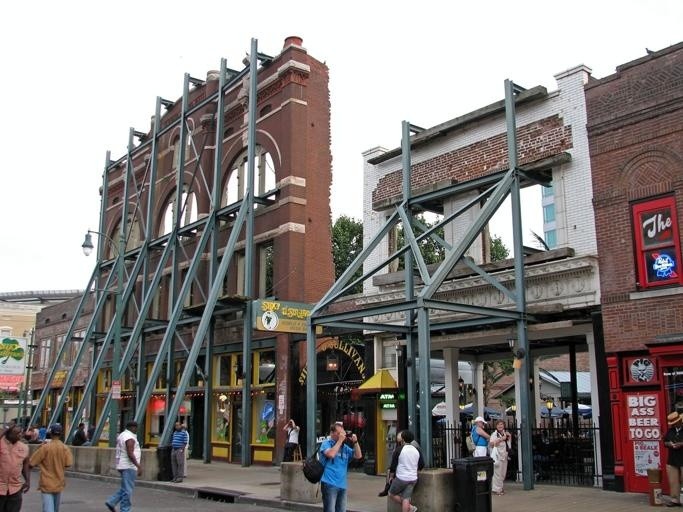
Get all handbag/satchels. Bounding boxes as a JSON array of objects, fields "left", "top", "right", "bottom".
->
[{"left": 302, "top": 454, "right": 324, "bottom": 484}]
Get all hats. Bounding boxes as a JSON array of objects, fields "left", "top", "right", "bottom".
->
[
  {"left": 475, "top": 416, "right": 487, "bottom": 424},
  {"left": 44, "top": 423, "right": 63, "bottom": 434}
]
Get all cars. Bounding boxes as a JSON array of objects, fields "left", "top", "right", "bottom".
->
[{"left": 10, "top": 416, "right": 31, "bottom": 428}]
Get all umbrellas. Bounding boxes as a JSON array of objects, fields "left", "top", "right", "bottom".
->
[
  {"left": 485, "top": 406, "right": 501, "bottom": 415},
  {"left": 432, "top": 401, "right": 448, "bottom": 422},
  {"left": 435, "top": 417, "right": 447, "bottom": 427},
  {"left": 538, "top": 403, "right": 592, "bottom": 429},
  {"left": 462, "top": 403, "right": 486, "bottom": 413}
]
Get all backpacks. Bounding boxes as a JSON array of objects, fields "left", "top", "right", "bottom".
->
[{"left": 466, "top": 428, "right": 480, "bottom": 454}]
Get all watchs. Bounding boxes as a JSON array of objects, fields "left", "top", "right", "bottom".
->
[{"left": 351, "top": 440, "right": 358, "bottom": 445}]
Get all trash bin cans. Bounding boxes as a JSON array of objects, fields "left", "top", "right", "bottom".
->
[
  {"left": 451, "top": 456, "right": 495, "bottom": 512},
  {"left": 156, "top": 446, "right": 173, "bottom": 481}
]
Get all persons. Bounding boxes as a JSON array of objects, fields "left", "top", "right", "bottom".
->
[
  {"left": 471, "top": 416, "right": 490, "bottom": 482},
  {"left": 169, "top": 422, "right": 188, "bottom": 483},
  {"left": 180, "top": 424, "right": 190, "bottom": 478},
  {"left": 489, "top": 419, "right": 512, "bottom": 495},
  {"left": 263, "top": 311, "right": 272, "bottom": 329},
  {"left": 319, "top": 423, "right": 362, "bottom": 512},
  {"left": 283, "top": 416, "right": 300, "bottom": 462},
  {"left": 106, "top": 421, "right": 143, "bottom": 512},
  {"left": 663, "top": 412, "right": 683, "bottom": 506},
  {"left": 388, "top": 431, "right": 425, "bottom": 512},
  {"left": 378, "top": 432, "right": 422, "bottom": 497},
  {"left": 0, "top": 416, "right": 96, "bottom": 512}
]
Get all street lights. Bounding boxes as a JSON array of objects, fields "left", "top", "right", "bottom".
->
[
  {"left": 81, "top": 230, "right": 141, "bottom": 316},
  {"left": 433, "top": 376, "right": 465, "bottom": 393},
  {"left": 20, "top": 327, "right": 36, "bottom": 429}
]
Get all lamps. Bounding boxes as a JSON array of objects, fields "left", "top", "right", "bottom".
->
[
  {"left": 396, "top": 341, "right": 411, "bottom": 367},
  {"left": 233, "top": 363, "right": 245, "bottom": 379},
  {"left": 506, "top": 334, "right": 524, "bottom": 359}
]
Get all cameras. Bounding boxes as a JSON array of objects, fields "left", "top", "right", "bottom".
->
[{"left": 345, "top": 432, "right": 352, "bottom": 439}]
